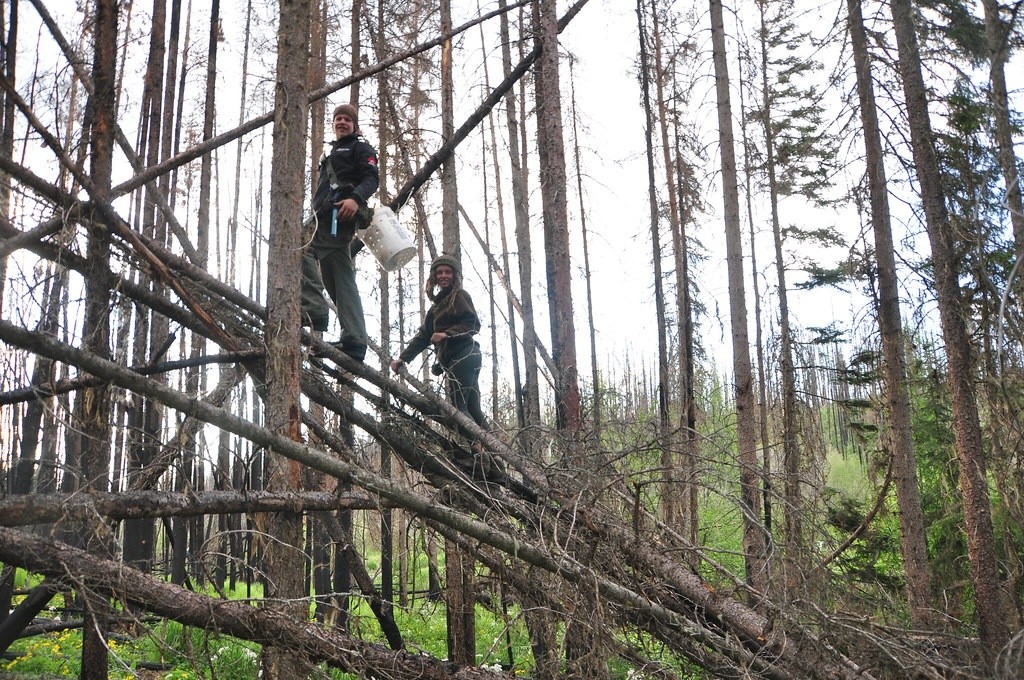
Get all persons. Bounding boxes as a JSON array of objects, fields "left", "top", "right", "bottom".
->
[
  {"left": 301, "top": 103, "right": 380, "bottom": 362},
  {"left": 390, "top": 254, "right": 505, "bottom": 481}
]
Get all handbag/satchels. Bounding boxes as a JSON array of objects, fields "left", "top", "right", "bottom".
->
[{"left": 327, "top": 182, "right": 374, "bottom": 229}]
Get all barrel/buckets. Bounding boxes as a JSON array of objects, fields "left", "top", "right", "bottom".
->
[{"left": 356, "top": 205, "right": 417, "bottom": 272}]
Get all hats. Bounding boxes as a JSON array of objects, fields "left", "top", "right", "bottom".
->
[
  {"left": 426, "top": 254, "right": 462, "bottom": 316},
  {"left": 333, "top": 105, "right": 358, "bottom": 124}
]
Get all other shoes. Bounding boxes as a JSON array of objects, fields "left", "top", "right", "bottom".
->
[
  {"left": 472, "top": 444, "right": 490, "bottom": 467},
  {"left": 491, "top": 459, "right": 506, "bottom": 472},
  {"left": 309, "top": 341, "right": 366, "bottom": 362},
  {"left": 300, "top": 313, "right": 328, "bottom": 331}
]
